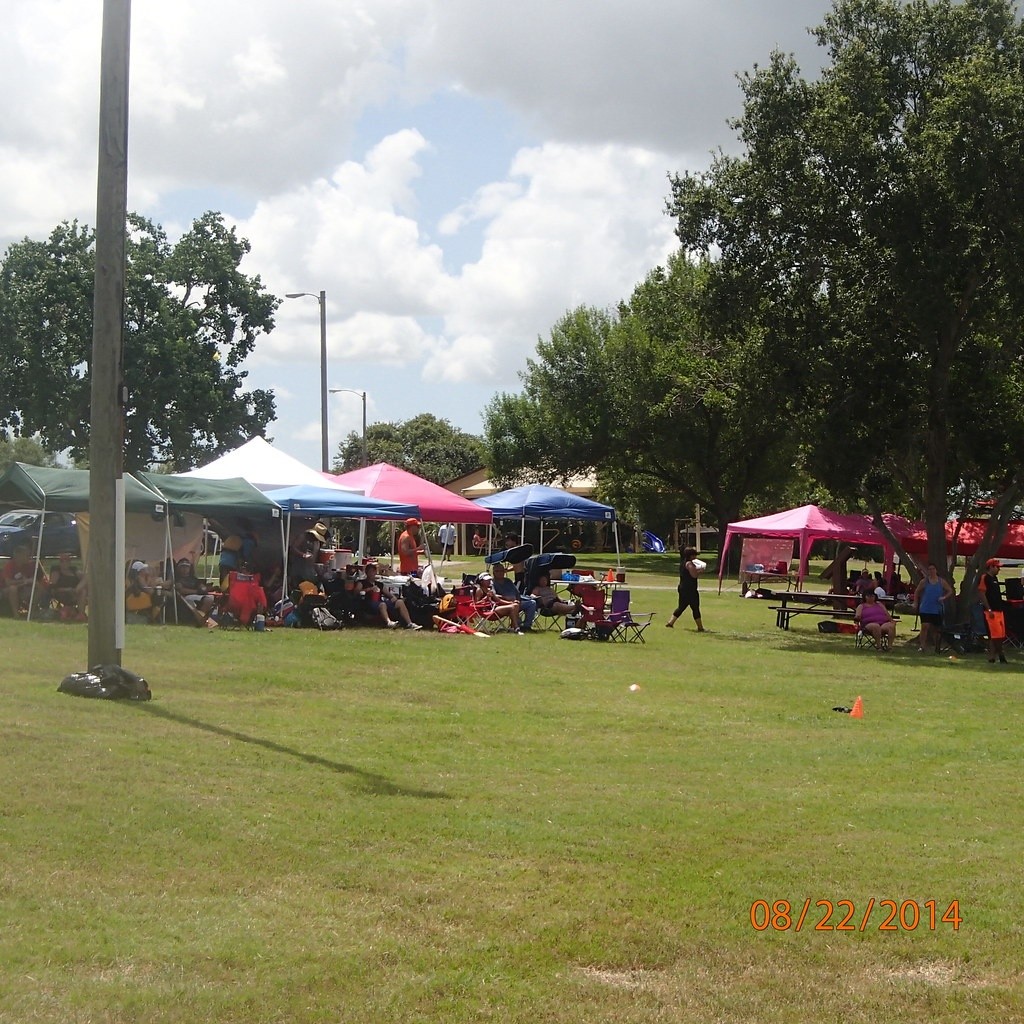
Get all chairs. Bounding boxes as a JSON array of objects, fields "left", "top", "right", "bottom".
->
[
  {"left": 935, "top": 605, "right": 970, "bottom": 656},
  {"left": 1001, "top": 578, "right": 1024, "bottom": 649},
  {"left": 125, "top": 559, "right": 260, "bottom": 631},
  {"left": 437, "top": 544, "right": 656, "bottom": 644}
]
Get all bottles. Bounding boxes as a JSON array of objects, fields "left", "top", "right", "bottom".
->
[
  {"left": 606, "top": 568, "right": 614, "bottom": 582},
  {"left": 563, "top": 571, "right": 571, "bottom": 581}
]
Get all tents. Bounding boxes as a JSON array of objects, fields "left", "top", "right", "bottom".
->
[
  {"left": 718, "top": 506, "right": 1024, "bottom": 615},
  {"left": 0, "top": 435, "right": 493, "bottom": 622},
  {"left": 474, "top": 484, "right": 620, "bottom": 567}
]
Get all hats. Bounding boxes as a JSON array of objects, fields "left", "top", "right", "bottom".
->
[
  {"left": 178, "top": 558, "right": 190, "bottom": 566},
  {"left": 307, "top": 523, "right": 327, "bottom": 543},
  {"left": 493, "top": 564, "right": 505, "bottom": 571},
  {"left": 405, "top": 518, "right": 421, "bottom": 525},
  {"left": 986, "top": 559, "right": 1004, "bottom": 567},
  {"left": 479, "top": 572, "right": 493, "bottom": 580},
  {"left": 132, "top": 561, "right": 149, "bottom": 569},
  {"left": 685, "top": 547, "right": 700, "bottom": 555},
  {"left": 60, "top": 553, "right": 71, "bottom": 559}
]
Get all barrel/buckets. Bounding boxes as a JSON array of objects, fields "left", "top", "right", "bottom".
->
[{"left": 334, "top": 549, "right": 353, "bottom": 568}]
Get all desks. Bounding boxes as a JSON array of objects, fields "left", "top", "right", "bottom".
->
[
  {"left": 743, "top": 570, "right": 800, "bottom": 592},
  {"left": 771, "top": 590, "right": 898, "bottom": 630},
  {"left": 544, "top": 579, "right": 628, "bottom": 630}
]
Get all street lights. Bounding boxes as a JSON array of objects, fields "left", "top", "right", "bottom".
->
[
  {"left": 329, "top": 389, "right": 367, "bottom": 468},
  {"left": 285, "top": 290, "right": 329, "bottom": 472}
]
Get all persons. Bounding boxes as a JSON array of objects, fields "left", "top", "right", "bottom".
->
[
  {"left": 126, "top": 561, "right": 162, "bottom": 618},
  {"left": 292, "top": 522, "right": 328, "bottom": 585},
  {"left": 980, "top": 559, "right": 1007, "bottom": 664},
  {"left": 438, "top": 522, "right": 456, "bottom": 561},
  {"left": 474, "top": 532, "right": 581, "bottom": 634},
  {"left": 314, "top": 564, "right": 330, "bottom": 595},
  {"left": 0, "top": 546, "right": 44, "bottom": 617},
  {"left": 398, "top": 518, "right": 426, "bottom": 596},
  {"left": 353, "top": 564, "right": 423, "bottom": 630},
  {"left": 219, "top": 533, "right": 258, "bottom": 586},
  {"left": 473, "top": 528, "right": 485, "bottom": 547},
  {"left": 913, "top": 563, "right": 953, "bottom": 656},
  {"left": 665, "top": 547, "right": 704, "bottom": 632},
  {"left": 49, "top": 553, "right": 88, "bottom": 621},
  {"left": 176, "top": 558, "right": 221, "bottom": 628},
  {"left": 854, "top": 562, "right": 906, "bottom": 652}
]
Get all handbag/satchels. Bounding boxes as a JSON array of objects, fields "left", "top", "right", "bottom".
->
[{"left": 984, "top": 610, "right": 1005, "bottom": 638}]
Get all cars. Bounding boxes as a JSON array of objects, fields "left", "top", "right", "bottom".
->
[{"left": 0, "top": 509, "right": 80, "bottom": 557}]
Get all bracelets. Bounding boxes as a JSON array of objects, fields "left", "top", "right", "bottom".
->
[
  {"left": 944, "top": 596, "right": 946, "bottom": 599},
  {"left": 988, "top": 609, "right": 991, "bottom": 612}
]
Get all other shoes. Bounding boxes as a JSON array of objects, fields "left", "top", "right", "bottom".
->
[
  {"left": 935, "top": 647, "right": 941, "bottom": 654},
  {"left": 698, "top": 628, "right": 704, "bottom": 632},
  {"left": 389, "top": 620, "right": 399, "bottom": 629},
  {"left": 878, "top": 647, "right": 894, "bottom": 654},
  {"left": 989, "top": 657, "right": 996, "bottom": 664},
  {"left": 999, "top": 655, "right": 1007, "bottom": 664},
  {"left": 665, "top": 622, "right": 673, "bottom": 628},
  {"left": 571, "top": 599, "right": 582, "bottom": 617},
  {"left": 408, "top": 622, "right": 423, "bottom": 630},
  {"left": 918, "top": 646, "right": 925, "bottom": 652}
]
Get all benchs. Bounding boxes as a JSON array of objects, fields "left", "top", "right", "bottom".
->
[{"left": 768, "top": 606, "right": 902, "bottom": 631}]
